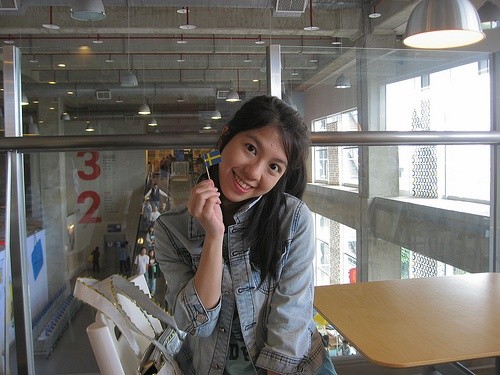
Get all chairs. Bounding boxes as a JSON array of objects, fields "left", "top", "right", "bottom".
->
[{"left": 86, "top": 274, "right": 164, "bottom": 375}]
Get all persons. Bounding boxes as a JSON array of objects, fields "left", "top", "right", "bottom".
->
[
  {"left": 91, "top": 246, "right": 100, "bottom": 273},
  {"left": 119, "top": 94, "right": 354, "bottom": 375}
]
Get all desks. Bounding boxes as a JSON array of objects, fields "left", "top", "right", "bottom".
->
[{"left": 313, "top": 272, "right": 500, "bottom": 375}]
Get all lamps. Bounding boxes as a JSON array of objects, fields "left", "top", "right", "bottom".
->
[
  {"left": 226, "top": 35, "right": 242, "bottom": 101},
  {"left": 333, "top": 4, "right": 352, "bottom": 88},
  {"left": 211, "top": 95, "right": 222, "bottom": 119},
  {"left": 61, "top": 100, "right": 70, "bottom": 120},
  {"left": 203, "top": 97, "right": 212, "bottom": 130},
  {"left": 85, "top": 101, "right": 94, "bottom": 131},
  {"left": 148, "top": 94, "right": 158, "bottom": 126},
  {"left": 402, "top": 0, "right": 485, "bottom": 50},
  {"left": 120, "top": 0, "right": 138, "bottom": 87},
  {"left": 71, "top": 0, "right": 106, "bottom": 22},
  {"left": 138, "top": 79, "right": 151, "bottom": 114}
]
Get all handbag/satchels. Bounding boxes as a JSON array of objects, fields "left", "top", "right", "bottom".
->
[{"left": 72, "top": 274, "right": 189, "bottom": 375}]
